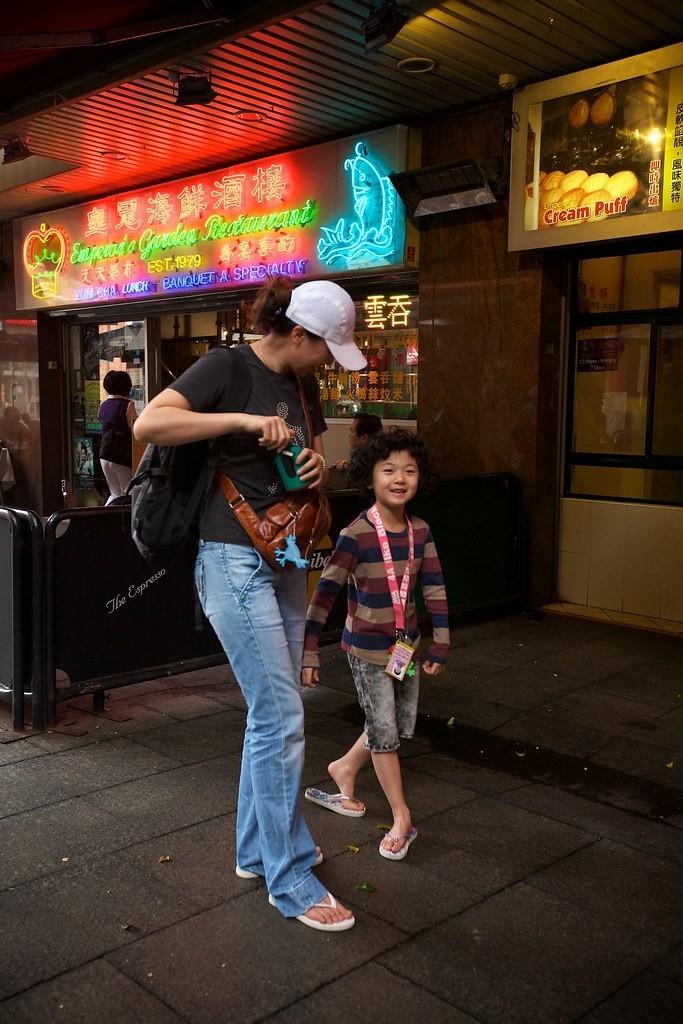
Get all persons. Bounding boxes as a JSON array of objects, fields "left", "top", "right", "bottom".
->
[
  {"left": 0, "top": 406, "right": 36, "bottom": 443},
  {"left": 301, "top": 423, "right": 450, "bottom": 860},
  {"left": 97, "top": 370, "right": 138, "bottom": 507},
  {"left": 132, "top": 276, "right": 368, "bottom": 931},
  {"left": 394, "top": 660, "right": 405, "bottom": 675},
  {"left": 325, "top": 413, "right": 383, "bottom": 549},
  {"left": 79, "top": 440, "right": 94, "bottom": 474}
]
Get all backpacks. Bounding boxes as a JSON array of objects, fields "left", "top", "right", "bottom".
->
[{"left": 124, "top": 346, "right": 251, "bottom": 573}]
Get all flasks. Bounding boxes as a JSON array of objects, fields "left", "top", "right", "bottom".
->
[{"left": 274, "top": 445, "right": 314, "bottom": 491}]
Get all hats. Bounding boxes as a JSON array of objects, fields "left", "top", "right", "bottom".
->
[{"left": 285, "top": 281, "right": 369, "bottom": 371}]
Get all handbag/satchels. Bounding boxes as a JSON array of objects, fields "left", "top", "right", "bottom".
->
[{"left": 255, "top": 488, "right": 331, "bottom": 571}]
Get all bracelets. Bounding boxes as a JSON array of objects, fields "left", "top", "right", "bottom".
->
[{"left": 315, "top": 453, "right": 325, "bottom": 471}]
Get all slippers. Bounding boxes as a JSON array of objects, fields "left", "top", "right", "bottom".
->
[
  {"left": 237, "top": 848, "right": 323, "bottom": 879},
  {"left": 303, "top": 788, "right": 367, "bottom": 817},
  {"left": 380, "top": 827, "right": 419, "bottom": 859},
  {"left": 269, "top": 892, "right": 354, "bottom": 932}
]
